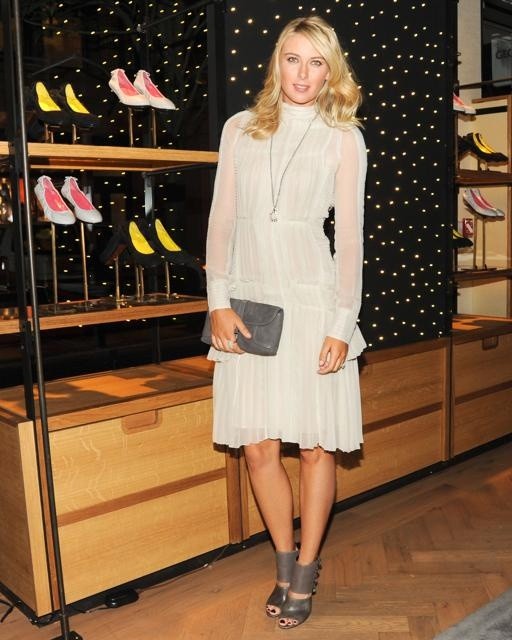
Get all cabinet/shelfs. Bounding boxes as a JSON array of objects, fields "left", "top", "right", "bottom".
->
[
  {"left": 454, "top": 94, "right": 511, "bottom": 286},
  {"left": 243, "top": 337, "right": 452, "bottom": 545},
  {"left": 450, "top": 311, "right": 512, "bottom": 465},
  {"left": 0, "top": 364, "right": 241, "bottom": 629},
  {"left": 0, "top": 138, "right": 223, "bottom": 337}
]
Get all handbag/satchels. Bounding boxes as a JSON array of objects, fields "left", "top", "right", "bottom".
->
[{"left": 200, "top": 298, "right": 283, "bottom": 356}]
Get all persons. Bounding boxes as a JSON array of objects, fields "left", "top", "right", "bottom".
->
[{"left": 205, "top": 15, "right": 368, "bottom": 629}]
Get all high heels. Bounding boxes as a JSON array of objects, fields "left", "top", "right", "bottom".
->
[
  {"left": 452, "top": 92, "right": 476, "bottom": 114},
  {"left": 122, "top": 217, "right": 190, "bottom": 264},
  {"left": 34, "top": 175, "right": 103, "bottom": 225},
  {"left": 265, "top": 546, "right": 320, "bottom": 629},
  {"left": 467, "top": 132, "right": 509, "bottom": 162},
  {"left": 448, "top": 229, "right": 473, "bottom": 248},
  {"left": 462, "top": 188, "right": 505, "bottom": 217},
  {"left": 33, "top": 80, "right": 100, "bottom": 125},
  {"left": 108, "top": 68, "right": 176, "bottom": 110}
]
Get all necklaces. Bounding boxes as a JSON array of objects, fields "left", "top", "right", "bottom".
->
[{"left": 270, "top": 112, "right": 319, "bottom": 224}]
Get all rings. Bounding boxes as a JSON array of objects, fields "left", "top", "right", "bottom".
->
[
  {"left": 340, "top": 365, "right": 345, "bottom": 369},
  {"left": 227, "top": 341, "right": 232, "bottom": 348}
]
